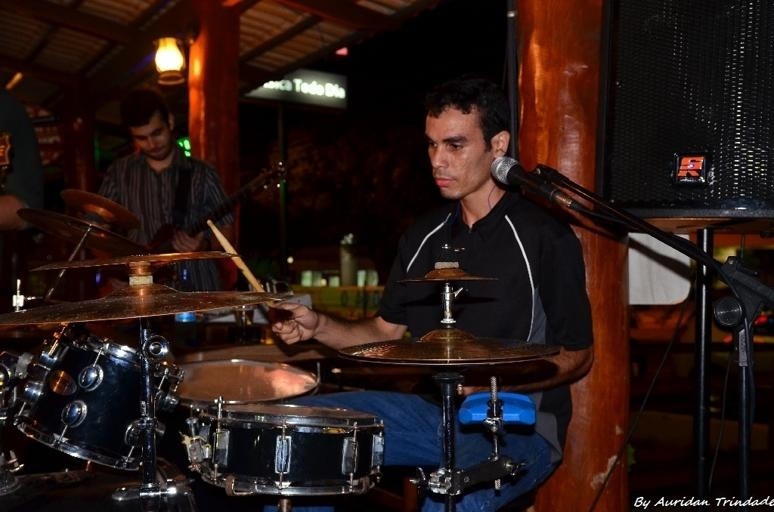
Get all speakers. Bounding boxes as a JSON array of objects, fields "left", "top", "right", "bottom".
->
[{"left": 594, "top": 0, "right": 774, "bottom": 218}]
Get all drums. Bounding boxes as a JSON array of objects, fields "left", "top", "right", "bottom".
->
[
  {"left": 10, "top": 321, "right": 178, "bottom": 473},
  {"left": 179, "top": 402, "right": 385, "bottom": 499}
]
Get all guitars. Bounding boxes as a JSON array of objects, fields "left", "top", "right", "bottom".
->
[{"left": 149, "top": 156, "right": 316, "bottom": 289}]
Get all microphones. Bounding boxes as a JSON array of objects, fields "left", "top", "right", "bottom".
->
[{"left": 490, "top": 157, "right": 579, "bottom": 209}]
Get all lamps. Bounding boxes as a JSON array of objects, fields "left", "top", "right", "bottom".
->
[{"left": 152, "top": 21, "right": 186, "bottom": 86}]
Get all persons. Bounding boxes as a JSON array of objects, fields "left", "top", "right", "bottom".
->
[
  {"left": 0, "top": 79, "right": 48, "bottom": 232},
  {"left": 265, "top": 73, "right": 596, "bottom": 512},
  {"left": 98, "top": 82, "right": 234, "bottom": 291}
]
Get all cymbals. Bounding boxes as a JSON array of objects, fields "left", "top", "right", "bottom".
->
[
  {"left": 386, "top": 271, "right": 507, "bottom": 302},
  {"left": 64, "top": 189, "right": 141, "bottom": 237},
  {"left": 174, "top": 332, "right": 332, "bottom": 367},
  {"left": 338, "top": 325, "right": 563, "bottom": 386},
  {"left": 27, "top": 250, "right": 238, "bottom": 277},
  {"left": 18, "top": 207, "right": 151, "bottom": 255},
  {"left": 0, "top": 282, "right": 298, "bottom": 328},
  {"left": 160, "top": 358, "right": 320, "bottom": 404}
]
